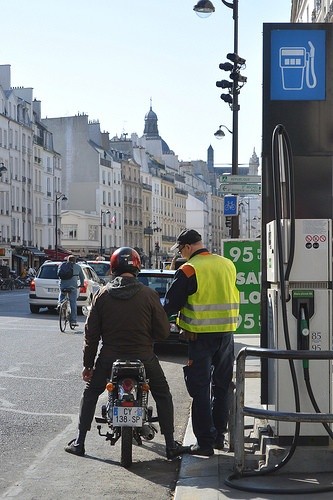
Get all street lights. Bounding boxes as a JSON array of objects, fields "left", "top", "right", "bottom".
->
[
  {"left": 100, "top": 209, "right": 111, "bottom": 256},
  {"left": 193, "top": 0, "right": 247, "bottom": 240},
  {"left": 55, "top": 194, "right": 68, "bottom": 262},
  {"left": 149, "top": 220, "right": 157, "bottom": 282}
]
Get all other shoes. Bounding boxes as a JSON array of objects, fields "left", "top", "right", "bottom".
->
[
  {"left": 64, "top": 438, "right": 85, "bottom": 456},
  {"left": 213, "top": 440, "right": 225, "bottom": 449},
  {"left": 72, "top": 323, "right": 79, "bottom": 326},
  {"left": 166, "top": 440, "right": 183, "bottom": 459},
  {"left": 58, "top": 306, "right": 60, "bottom": 311},
  {"left": 188, "top": 442, "right": 214, "bottom": 456}
]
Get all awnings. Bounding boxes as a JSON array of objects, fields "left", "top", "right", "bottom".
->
[
  {"left": 44, "top": 248, "right": 67, "bottom": 259},
  {"left": 17, "top": 245, "right": 46, "bottom": 256},
  {"left": 13, "top": 254, "right": 29, "bottom": 260}
]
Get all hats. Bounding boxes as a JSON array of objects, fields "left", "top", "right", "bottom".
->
[{"left": 170, "top": 229, "right": 201, "bottom": 251}]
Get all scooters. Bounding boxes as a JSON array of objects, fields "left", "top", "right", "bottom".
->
[{"left": 0, "top": 271, "right": 35, "bottom": 290}]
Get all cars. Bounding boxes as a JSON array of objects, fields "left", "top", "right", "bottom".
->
[
  {"left": 137, "top": 269, "right": 189, "bottom": 357},
  {"left": 28, "top": 260, "right": 105, "bottom": 316},
  {"left": 86, "top": 257, "right": 113, "bottom": 286}
]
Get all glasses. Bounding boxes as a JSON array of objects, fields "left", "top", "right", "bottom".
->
[{"left": 177, "top": 246, "right": 186, "bottom": 254}]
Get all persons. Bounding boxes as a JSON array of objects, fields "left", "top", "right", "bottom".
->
[
  {"left": 21, "top": 266, "right": 36, "bottom": 276},
  {"left": 161, "top": 228, "right": 236, "bottom": 457},
  {"left": 59, "top": 254, "right": 85, "bottom": 326},
  {"left": 64, "top": 247, "right": 191, "bottom": 455}
]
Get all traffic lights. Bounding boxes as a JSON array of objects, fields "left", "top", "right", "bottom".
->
[{"left": 225, "top": 216, "right": 238, "bottom": 238}]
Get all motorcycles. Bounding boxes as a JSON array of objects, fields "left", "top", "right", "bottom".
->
[{"left": 95, "top": 357, "right": 160, "bottom": 468}]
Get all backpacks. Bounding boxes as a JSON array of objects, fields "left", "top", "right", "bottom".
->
[{"left": 57, "top": 261, "right": 79, "bottom": 280}]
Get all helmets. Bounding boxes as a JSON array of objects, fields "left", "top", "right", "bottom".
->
[{"left": 110, "top": 247, "right": 141, "bottom": 278}]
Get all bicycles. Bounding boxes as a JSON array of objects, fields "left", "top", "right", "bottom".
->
[{"left": 58, "top": 285, "right": 85, "bottom": 332}]
